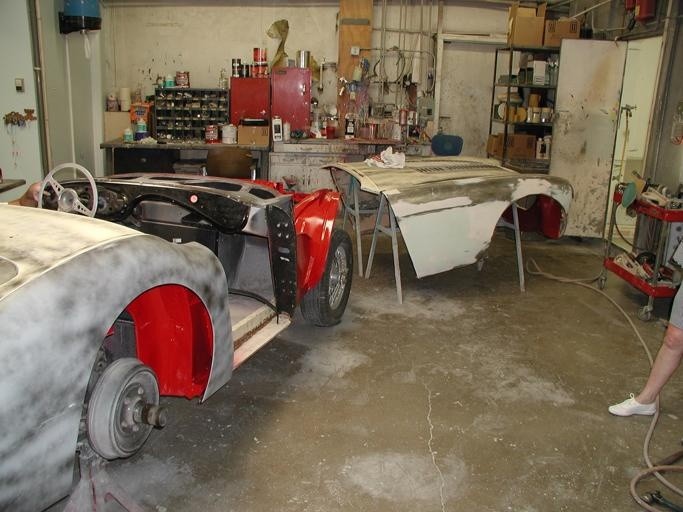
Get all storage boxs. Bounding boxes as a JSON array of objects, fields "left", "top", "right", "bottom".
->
[
  {"left": 544, "top": 19, "right": 581, "bottom": 47},
  {"left": 485, "top": 135, "right": 499, "bottom": 157},
  {"left": 507, "top": 0, "right": 547, "bottom": 50},
  {"left": 498, "top": 133, "right": 537, "bottom": 160}
]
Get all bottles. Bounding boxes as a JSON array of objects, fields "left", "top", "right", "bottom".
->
[
  {"left": 511, "top": 75, "right": 517, "bottom": 83},
  {"left": 519, "top": 68, "right": 526, "bottom": 84},
  {"left": 527, "top": 67, "right": 533, "bottom": 84},
  {"left": 284, "top": 120, "right": 290, "bottom": 140}
]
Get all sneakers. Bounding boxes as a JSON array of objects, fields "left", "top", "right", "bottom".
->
[{"left": 609, "top": 399, "right": 656, "bottom": 416}]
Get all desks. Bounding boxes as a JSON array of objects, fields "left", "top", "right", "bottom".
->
[{"left": 100, "top": 136, "right": 270, "bottom": 192}]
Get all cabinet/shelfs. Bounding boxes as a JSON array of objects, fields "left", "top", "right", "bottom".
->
[
  {"left": 231, "top": 67, "right": 310, "bottom": 137},
  {"left": 152, "top": 89, "right": 230, "bottom": 140},
  {"left": 599, "top": 182, "right": 683, "bottom": 321},
  {"left": 487, "top": 48, "right": 566, "bottom": 171},
  {"left": 271, "top": 152, "right": 349, "bottom": 205}
]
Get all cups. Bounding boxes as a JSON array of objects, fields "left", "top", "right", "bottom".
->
[{"left": 326, "top": 127, "right": 335, "bottom": 140}]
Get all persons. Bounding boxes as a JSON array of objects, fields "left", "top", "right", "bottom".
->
[
  {"left": 608, "top": 235, "right": 683, "bottom": 416},
  {"left": 0, "top": 181, "right": 52, "bottom": 208}
]
